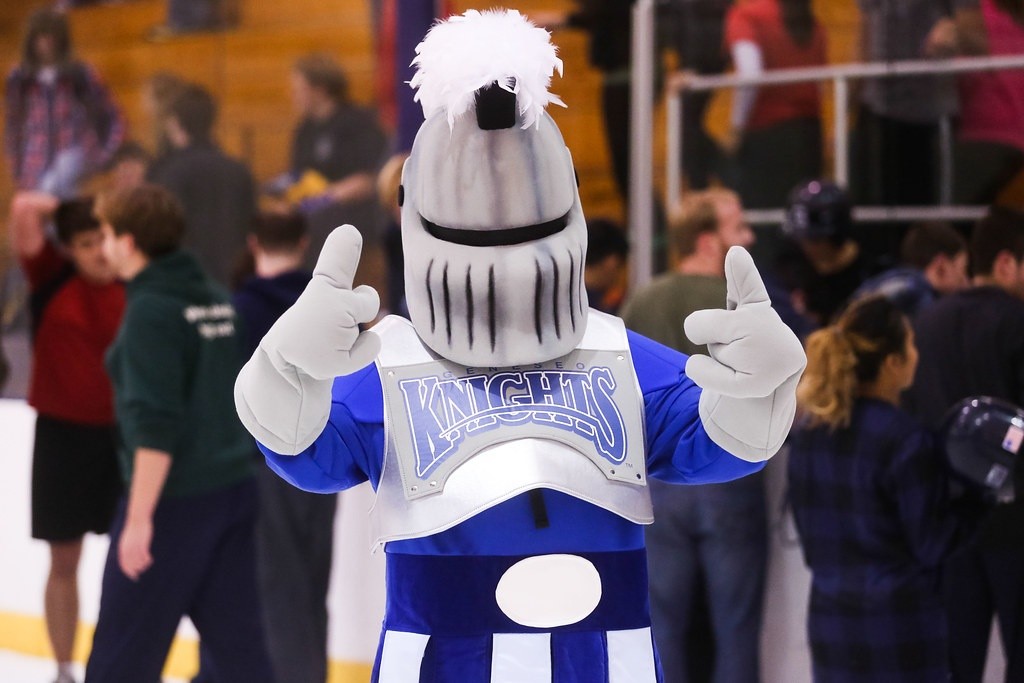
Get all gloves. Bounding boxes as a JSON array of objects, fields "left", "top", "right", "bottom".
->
[
  {"left": 234, "top": 224, "right": 382, "bottom": 455},
  {"left": 684, "top": 245, "right": 808, "bottom": 463}
]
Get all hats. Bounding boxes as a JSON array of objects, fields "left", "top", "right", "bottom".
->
[
  {"left": 398, "top": 8, "right": 589, "bottom": 368},
  {"left": 783, "top": 183, "right": 851, "bottom": 220}
]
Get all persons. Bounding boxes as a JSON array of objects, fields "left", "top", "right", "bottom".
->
[
  {"left": 10, "top": 192, "right": 124, "bottom": 683},
  {"left": 232, "top": 202, "right": 338, "bottom": 683},
  {"left": 0, "top": 10, "right": 403, "bottom": 323},
  {"left": 233, "top": 100, "right": 807, "bottom": 683},
  {"left": 536, "top": 1, "right": 1024, "bottom": 683},
  {"left": 90, "top": 186, "right": 268, "bottom": 683}
]
